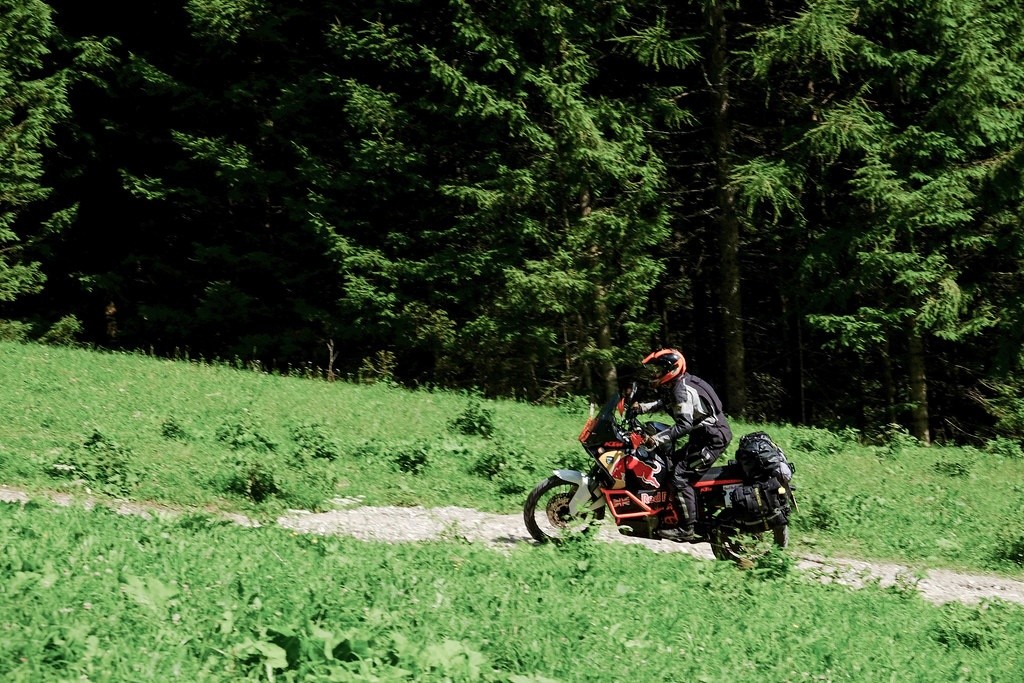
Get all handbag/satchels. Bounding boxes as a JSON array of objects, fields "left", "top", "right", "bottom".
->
[{"left": 730, "top": 430, "right": 797, "bottom": 535}]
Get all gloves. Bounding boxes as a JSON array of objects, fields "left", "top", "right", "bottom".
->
[
  {"left": 643, "top": 435, "right": 658, "bottom": 452},
  {"left": 630, "top": 401, "right": 642, "bottom": 415}
]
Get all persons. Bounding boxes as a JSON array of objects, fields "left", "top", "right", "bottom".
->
[{"left": 630, "top": 348, "right": 732, "bottom": 542}]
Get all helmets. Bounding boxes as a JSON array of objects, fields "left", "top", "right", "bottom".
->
[{"left": 641, "top": 348, "right": 686, "bottom": 391}]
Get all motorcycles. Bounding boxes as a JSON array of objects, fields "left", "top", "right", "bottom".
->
[{"left": 524, "top": 383, "right": 799, "bottom": 570}]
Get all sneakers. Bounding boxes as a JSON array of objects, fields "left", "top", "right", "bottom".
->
[{"left": 657, "top": 526, "right": 695, "bottom": 542}]
